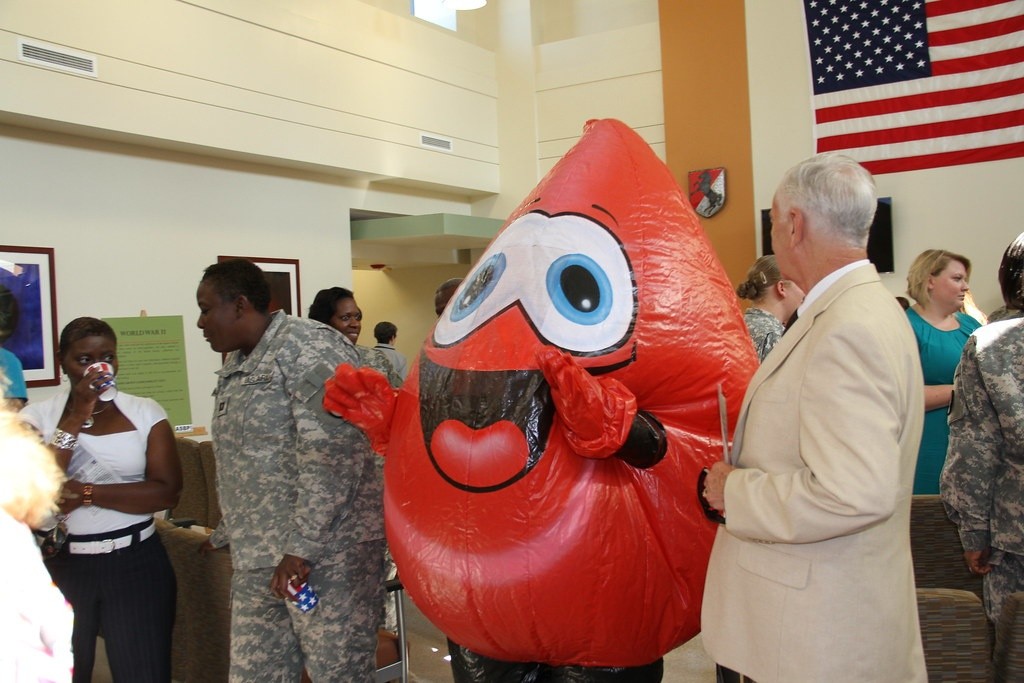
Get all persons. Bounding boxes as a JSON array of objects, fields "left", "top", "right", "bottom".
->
[
  {"left": 15, "top": 315, "right": 184, "bottom": 683},
  {"left": 0, "top": 283, "right": 29, "bottom": 414},
  {"left": 0, "top": 363, "right": 75, "bottom": 683},
  {"left": 701, "top": 152, "right": 929, "bottom": 683},
  {"left": 735, "top": 255, "right": 805, "bottom": 363},
  {"left": 374, "top": 322, "right": 406, "bottom": 383},
  {"left": 434, "top": 278, "right": 464, "bottom": 318},
  {"left": 903, "top": 249, "right": 988, "bottom": 494},
  {"left": 939, "top": 230, "right": 1024, "bottom": 683},
  {"left": 307, "top": 285, "right": 419, "bottom": 683},
  {"left": 197, "top": 259, "right": 391, "bottom": 683}
]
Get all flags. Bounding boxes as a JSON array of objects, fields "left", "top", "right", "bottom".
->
[{"left": 803, "top": 0, "right": 1024, "bottom": 176}]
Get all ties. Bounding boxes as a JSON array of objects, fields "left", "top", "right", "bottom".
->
[{"left": 781, "top": 308, "right": 798, "bottom": 336}]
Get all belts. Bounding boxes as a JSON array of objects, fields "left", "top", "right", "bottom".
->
[{"left": 69, "top": 522, "right": 156, "bottom": 555}]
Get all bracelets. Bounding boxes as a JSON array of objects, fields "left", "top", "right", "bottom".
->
[
  {"left": 83, "top": 482, "right": 93, "bottom": 507},
  {"left": 49, "top": 427, "right": 77, "bottom": 451}
]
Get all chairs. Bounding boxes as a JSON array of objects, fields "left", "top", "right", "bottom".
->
[
  {"left": 153, "top": 517, "right": 412, "bottom": 683},
  {"left": 993, "top": 592, "right": 1024, "bottom": 683},
  {"left": 165, "top": 437, "right": 208, "bottom": 526},
  {"left": 198, "top": 441, "right": 226, "bottom": 530},
  {"left": 915, "top": 586, "right": 990, "bottom": 682},
  {"left": 911, "top": 493, "right": 984, "bottom": 593}
]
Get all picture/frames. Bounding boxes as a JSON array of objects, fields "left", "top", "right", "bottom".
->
[
  {"left": 216, "top": 254, "right": 304, "bottom": 367},
  {"left": 0, "top": 243, "right": 61, "bottom": 389}
]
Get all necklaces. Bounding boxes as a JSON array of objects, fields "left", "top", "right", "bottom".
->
[{"left": 82, "top": 401, "right": 111, "bottom": 428}]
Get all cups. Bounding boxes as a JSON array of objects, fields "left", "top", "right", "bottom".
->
[
  {"left": 83, "top": 361, "right": 118, "bottom": 402},
  {"left": 277, "top": 577, "right": 319, "bottom": 614}
]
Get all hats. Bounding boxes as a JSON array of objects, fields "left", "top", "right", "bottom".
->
[{"left": 0, "top": 348, "right": 28, "bottom": 402}]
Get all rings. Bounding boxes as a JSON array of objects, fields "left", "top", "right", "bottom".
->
[
  {"left": 969, "top": 566, "right": 973, "bottom": 572},
  {"left": 703, "top": 488, "right": 708, "bottom": 497},
  {"left": 89, "top": 384, "right": 95, "bottom": 390}
]
